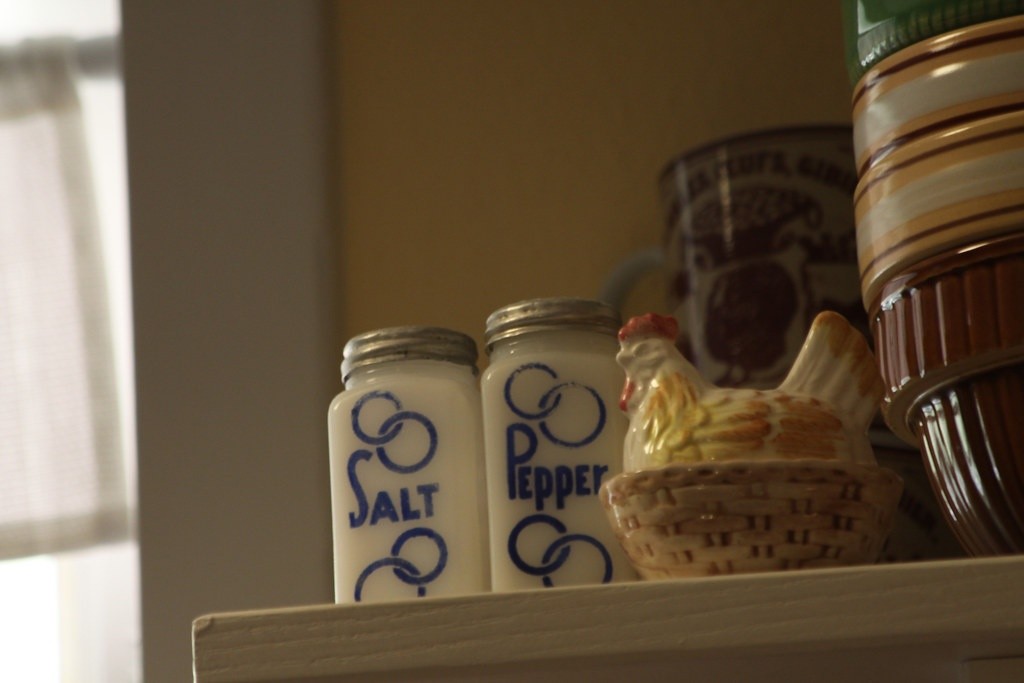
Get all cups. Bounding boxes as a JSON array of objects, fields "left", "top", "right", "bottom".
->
[
  {"left": 329, "top": 325, "right": 489, "bottom": 604},
  {"left": 600, "top": 121, "right": 948, "bottom": 561},
  {"left": 480, "top": 296, "right": 635, "bottom": 592}
]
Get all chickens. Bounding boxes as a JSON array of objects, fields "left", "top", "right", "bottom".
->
[{"left": 616, "top": 310, "right": 887, "bottom": 473}]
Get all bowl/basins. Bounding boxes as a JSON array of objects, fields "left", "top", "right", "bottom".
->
[{"left": 845, "top": 0, "right": 1024, "bottom": 557}]
